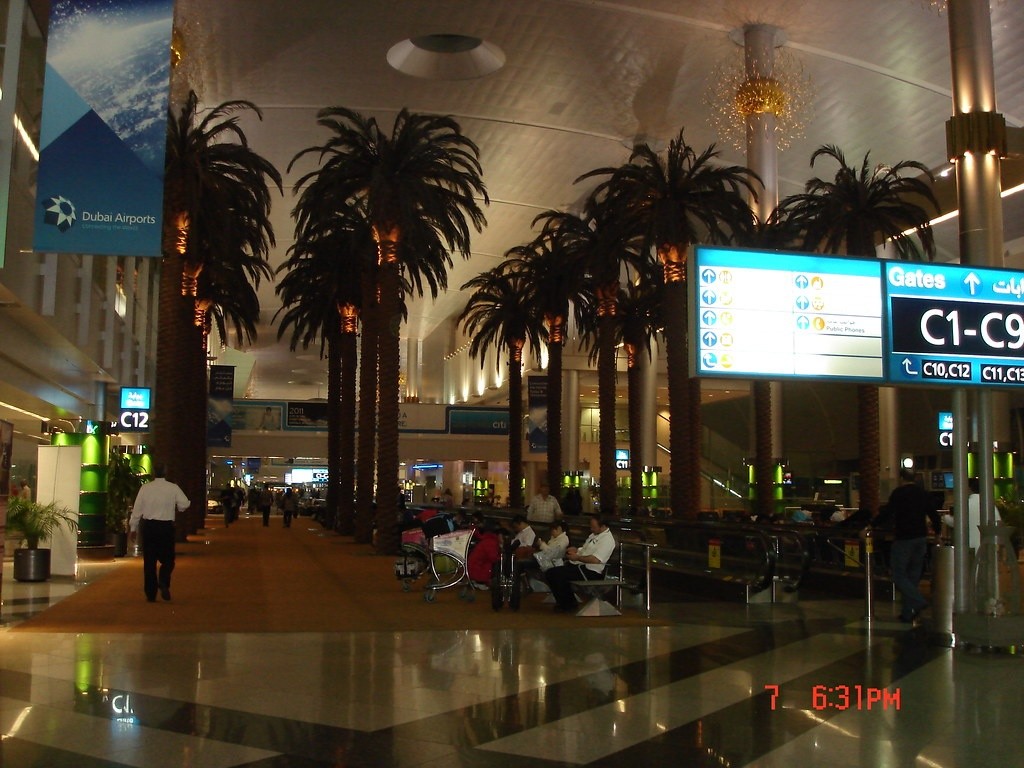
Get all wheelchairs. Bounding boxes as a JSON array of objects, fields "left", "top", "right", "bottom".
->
[{"left": 489, "top": 536, "right": 523, "bottom": 610}]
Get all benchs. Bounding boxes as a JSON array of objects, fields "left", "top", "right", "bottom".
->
[{"left": 529, "top": 562, "right": 629, "bottom": 617}]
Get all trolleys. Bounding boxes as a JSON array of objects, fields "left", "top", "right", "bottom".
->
[
  {"left": 394, "top": 523, "right": 435, "bottom": 593},
  {"left": 419, "top": 522, "right": 478, "bottom": 605}
]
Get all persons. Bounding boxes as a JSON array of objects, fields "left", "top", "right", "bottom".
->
[
  {"left": 398, "top": 479, "right": 617, "bottom": 612},
  {"left": 941, "top": 477, "right": 1002, "bottom": 588},
  {"left": 871, "top": 467, "right": 943, "bottom": 622},
  {"left": 19, "top": 479, "right": 31, "bottom": 501},
  {"left": 128, "top": 463, "right": 191, "bottom": 602},
  {"left": 219, "top": 482, "right": 321, "bottom": 528}
]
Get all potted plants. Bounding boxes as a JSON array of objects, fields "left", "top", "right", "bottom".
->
[
  {"left": 6, "top": 495, "right": 81, "bottom": 584},
  {"left": 105, "top": 447, "right": 153, "bottom": 558}
]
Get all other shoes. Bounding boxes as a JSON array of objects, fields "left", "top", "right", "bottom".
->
[
  {"left": 474, "top": 583, "right": 484, "bottom": 588},
  {"left": 898, "top": 610, "right": 915, "bottom": 621},
  {"left": 478, "top": 585, "right": 490, "bottom": 590},
  {"left": 911, "top": 598, "right": 927, "bottom": 618},
  {"left": 158, "top": 585, "right": 171, "bottom": 601},
  {"left": 147, "top": 595, "right": 157, "bottom": 602}
]
